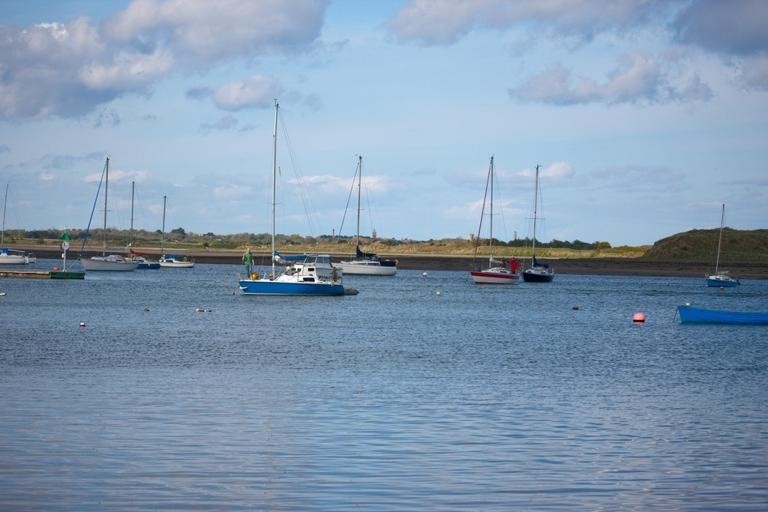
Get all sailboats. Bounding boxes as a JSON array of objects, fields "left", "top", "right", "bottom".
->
[
  {"left": 238, "top": 98, "right": 399, "bottom": 295},
  {"left": 0, "top": 157, "right": 194, "bottom": 279},
  {"left": 705, "top": 204, "right": 738, "bottom": 287},
  {"left": 470, "top": 156, "right": 554, "bottom": 283}
]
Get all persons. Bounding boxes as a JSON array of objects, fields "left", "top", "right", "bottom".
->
[
  {"left": 510, "top": 255, "right": 519, "bottom": 274},
  {"left": 128, "top": 247, "right": 136, "bottom": 261},
  {"left": 240, "top": 246, "right": 256, "bottom": 279}
]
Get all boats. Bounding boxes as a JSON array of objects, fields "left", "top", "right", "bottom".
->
[{"left": 672, "top": 304, "right": 768, "bottom": 326}]
